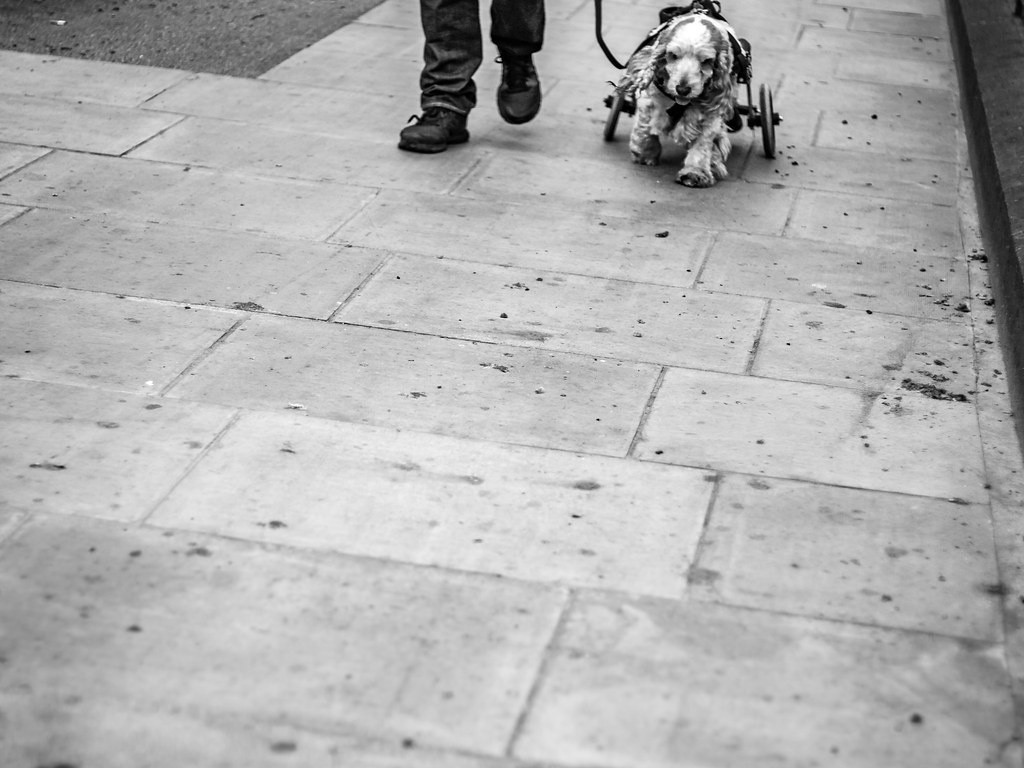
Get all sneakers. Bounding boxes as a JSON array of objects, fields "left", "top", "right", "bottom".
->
[
  {"left": 398, "top": 106, "right": 469, "bottom": 154},
  {"left": 494, "top": 49, "right": 541, "bottom": 125}
]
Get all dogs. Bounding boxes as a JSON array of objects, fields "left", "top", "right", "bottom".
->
[{"left": 614, "top": 10, "right": 749, "bottom": 188}]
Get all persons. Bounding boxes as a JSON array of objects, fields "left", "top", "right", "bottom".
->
[{"left": 398, "top": 0, "right": 546, "bottom": 154}]
